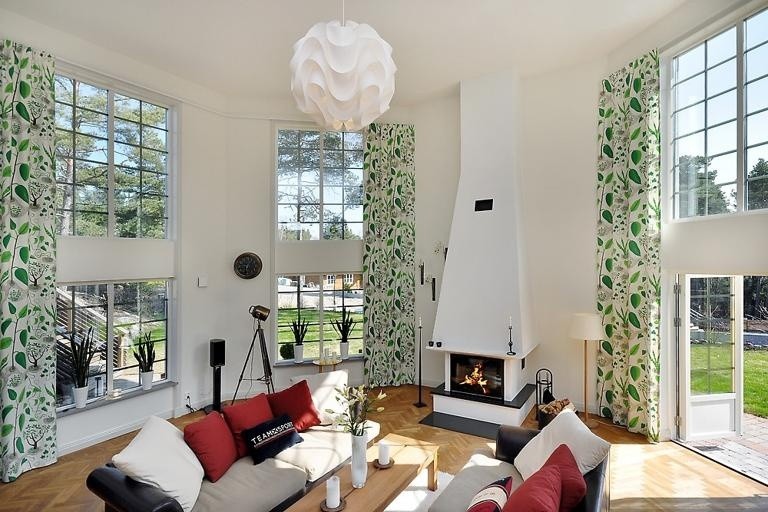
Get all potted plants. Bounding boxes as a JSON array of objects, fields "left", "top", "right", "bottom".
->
[
  {"left": 68, "top": 327, "right": 96, "bottom": 408},
  {"left": 331, "top": 307, "right": 357, "bottom": 359},
  {"left": 287, "top": 318, "right": 310, "bottom": 363},
  {"left": 131, "top": 332, "right": 155, "bottom": 390}
]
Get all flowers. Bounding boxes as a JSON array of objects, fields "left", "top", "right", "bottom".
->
[{"left": 325, "top": 383, "right": 387, "bottom": 435}]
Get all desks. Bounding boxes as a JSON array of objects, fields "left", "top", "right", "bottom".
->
[{"left": 313, "top": 358, "right": 343, "bottom": 374}]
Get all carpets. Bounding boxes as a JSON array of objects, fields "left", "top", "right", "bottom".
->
[{"left": 384, "top": 467, "right": 456, "bottom": 512}]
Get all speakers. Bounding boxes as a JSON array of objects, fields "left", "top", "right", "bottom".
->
[{"left": 209, "top": 338, "right": 226, "bottom": 367}]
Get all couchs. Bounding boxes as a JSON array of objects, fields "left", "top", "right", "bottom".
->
[
  {"left": 427, "top": 425, "right": 611, "bottom": 511},
  {"left": 87, "top": 415, "right": 380, "bottom": 512}
]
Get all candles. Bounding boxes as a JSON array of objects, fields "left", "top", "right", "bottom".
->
[
  {"left": 418, "top": 316, "right": 422, "bottom": 327},
  {"left": 325, "top": 474, "right": 342, "bottom": 508},
  {"left": 378, "top": 439, "right": 389, "bottom": 465}
]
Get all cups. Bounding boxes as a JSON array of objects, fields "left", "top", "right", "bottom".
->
[
  {"left": 320, "top": 353, "right": 324, "bottom": 359},
  {"left": 428, "top": 341, "right": 434, "bottom": 347},
  {"left": 325, "top": 348, "right": 329, "bottom": 359},
  {"left": 332, "top": 352, "right": 337, "bottom": 360},
  {"left": 436, "top": 342, "right": 442, "bottom": 347}
]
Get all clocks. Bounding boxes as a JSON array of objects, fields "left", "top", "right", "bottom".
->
[
  {"left": 234, "top": 252, "right": 262, "bottom": 280},
  {"left": 509, "top": 315, "right": 511, "bottom": 328}
]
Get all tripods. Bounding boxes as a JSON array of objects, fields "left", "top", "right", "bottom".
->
[{"left": 230, "top": 329, "right": 275, "bottom": 405}]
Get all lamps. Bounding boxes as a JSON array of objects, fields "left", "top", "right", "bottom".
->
[
  {"left": 286, "top": 0, "right": 397, "bottom": 132},
  {"left": 564, "top": 312, "right": 605, "bottom": 429}
]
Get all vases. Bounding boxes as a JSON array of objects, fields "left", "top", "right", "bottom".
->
[{"left": 350, "top": 430, "right": 367, "bottom": 488}]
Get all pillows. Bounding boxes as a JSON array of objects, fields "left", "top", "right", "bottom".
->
[
  {"left": 465, "top": 406, "right": 611, "bottom": 512},
  {"left": 111, "top": 367, "right": 349, "bottom": 512}
]
